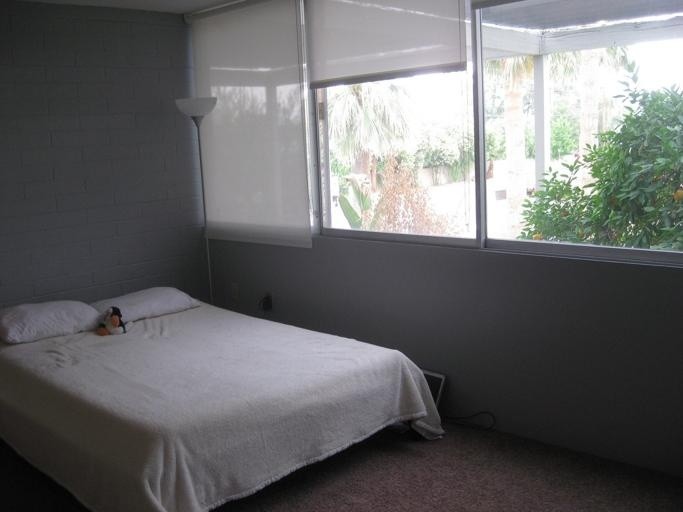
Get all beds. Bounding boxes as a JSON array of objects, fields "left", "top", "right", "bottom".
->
[{"left": 1, "top": 299, "right": 426, "bottom": 512}]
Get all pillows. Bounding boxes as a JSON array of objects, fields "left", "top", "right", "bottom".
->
[{"left": 0, "top": 286, "right": 202, "bottom": 346}]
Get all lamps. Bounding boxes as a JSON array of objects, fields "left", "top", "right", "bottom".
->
[{"left": 176, "top": 97, "right": 218, "bottom": 304}]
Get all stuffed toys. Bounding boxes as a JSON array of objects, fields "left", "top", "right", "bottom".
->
[{"left": 96, "top": 306, "right": 126, "bottom": 336}]
